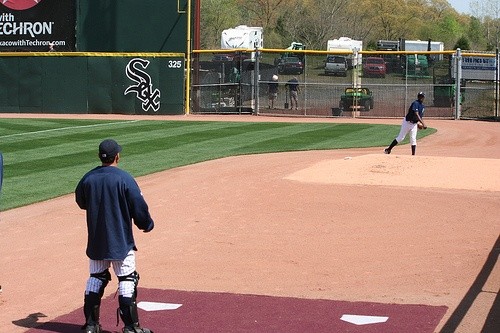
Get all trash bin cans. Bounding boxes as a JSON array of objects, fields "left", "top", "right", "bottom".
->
[{"left": 332, "top": 107, "right": 345, "bottom": 116}]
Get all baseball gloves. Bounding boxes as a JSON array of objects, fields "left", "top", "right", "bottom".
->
[{"left": 418, "top": 122, "right": 423, "bottom": 129}]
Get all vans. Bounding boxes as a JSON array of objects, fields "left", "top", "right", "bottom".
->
[{"left": 400, "top": 55, "right": 429, "bottom": 79}]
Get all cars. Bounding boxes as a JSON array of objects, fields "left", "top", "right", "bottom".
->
[
  {"left": 277, "top": 57, "right": 304, "bottom": 75},
  {"left": 339, "top": 87, "right": 374, "bottom": 111},
  {"left": 362, "top": 57, "right": 386, "bottom": 78}
]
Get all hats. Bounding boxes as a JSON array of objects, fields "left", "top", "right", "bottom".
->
[{"left": 99, "top": 139, "right": 120, "bottom": 158}]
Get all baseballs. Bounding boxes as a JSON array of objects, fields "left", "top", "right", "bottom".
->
[{"left": 271, "top": 75, "right": 278, "bottom": 81}]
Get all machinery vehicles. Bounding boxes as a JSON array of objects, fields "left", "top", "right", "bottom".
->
[{"left": 433, "top": 60, "right": 465, "bottom": 106}]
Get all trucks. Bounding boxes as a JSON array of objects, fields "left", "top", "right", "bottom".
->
[
  {"left": 327, "top": 36, "right": 362, "bottom": 68},
  {"left": 221, "top": 26, "right": 264, "bottom": 62},
  {"left": 374, "top": 38, "right": 443, "bottom": 63}
]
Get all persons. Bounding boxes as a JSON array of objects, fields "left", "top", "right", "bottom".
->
[
  {"left": 75, "top": 138, "right": 154, "bottom": 333},
  {"left": 284, "top": 77, "right": 300, "bottom": 110},
  {"left": 383, "top": 91, "right": 427, "bottom": 155},
  {"left": 0, "top": 152, "right": 3, "bottom": 291},
  {"left": 268, "top": 73, "right": 279, "bottom": 109}
]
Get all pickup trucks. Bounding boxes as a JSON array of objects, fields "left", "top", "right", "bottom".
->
[{"left": 323, "top": 55, "right": 348, "bottom": 76}]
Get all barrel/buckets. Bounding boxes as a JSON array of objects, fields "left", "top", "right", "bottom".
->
[
  {"left": 351, "top": 106, "right": 360, "bottom": 117},
  {"left": 332, "top": 108, "right": 343, "bottom": 116}
]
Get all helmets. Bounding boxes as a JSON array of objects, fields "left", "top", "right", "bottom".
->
[
  {"left": 271, "top": 75, "right": 279, "bottom": 81},
  {"left": 417, "top": 91, "right": 425, "bottom": 97}
]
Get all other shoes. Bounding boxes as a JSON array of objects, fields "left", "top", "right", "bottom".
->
[
  {"left": 383, "top": 149, "right": 391, "bottom": 154},
  {"left": 124, "top": 326, "right": 153, "bottom": 333},
  {"left": 86, "top": 324, "right": 100, "bottom": 333}
]
